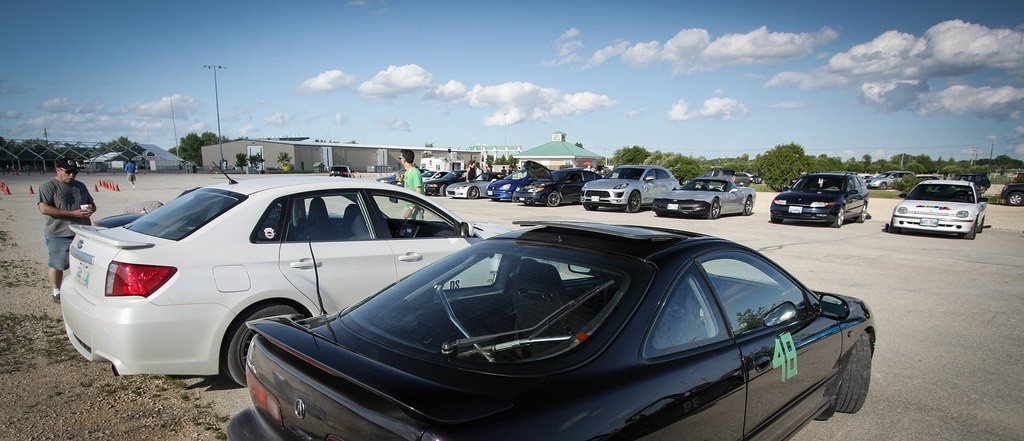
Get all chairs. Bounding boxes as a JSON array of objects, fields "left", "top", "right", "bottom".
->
[
  {"left": 508, "top": 258, "right": 569, "bottom": 348},
  {"left": 343, "top": 204, "right": 369, "bottom": 239},
  {"left": 307, "top": 198, "right": 330, "bottom": 240}
]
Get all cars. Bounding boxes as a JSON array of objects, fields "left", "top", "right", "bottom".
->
[
  {"left": 226, "top": 221, "right": 879, "bottom": 441},
  {"left": 512, "top": 169, "right": 603, "bottom": 208},
  {"left": 770, "top": 171, "right": 870, "bottom": 228},
  {"left": 375, "top": 166, "right": 468, "bottom": 197},
  {"left": 580, "top": 164, "right": 681, "bottom": 213},
  {"left": 330, "top": 166, "right": 352, "bottom": 177},
  {"left": 703, "top": 168, "right": 1024, "bottom": 206},
  {"left": 889, "top": 178, "right": 987, "bottom": 240},
  {"left": 60, "top": 162, "right": 562, "bottom": 388},
  {"left": 485, "top": 161, "right": 552, "bottom": 202},
  {"left": 445, "top": 172, "right": 508, "bottom": 199},
  {"left": 652, "top": 176, "right": 757, "bottom": 220}
]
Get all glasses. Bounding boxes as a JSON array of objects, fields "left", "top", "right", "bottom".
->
[
  {"left": 57, "top": 167, "right": 78, "bottom": 174},
  {"left": 399, "top": 156, "right": 404, "bottom": 160}
]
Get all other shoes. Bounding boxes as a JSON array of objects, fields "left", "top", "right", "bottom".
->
[{"left": 52, "top": 294, "right": 61, "bottom": 306}]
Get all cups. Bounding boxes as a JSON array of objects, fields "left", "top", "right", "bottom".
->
[{"left": 80, "top": 204, "right": 88, "bottom": 212}]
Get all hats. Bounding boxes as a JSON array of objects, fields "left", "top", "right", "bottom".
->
[{"left": 56, "top": 157, "right": 85, "bottom": 169}]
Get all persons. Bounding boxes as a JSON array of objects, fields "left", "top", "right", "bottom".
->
[
  {"left": 125, "top": 159, "right": 137, "bottom": 190},
  {"left": 501, "top": 166, "right": 507, "bottom": 177},
  {"left": 210, "top": 165, "right": 213, "bottom": 175},
  {"left": 508, "top": 166, "right": 513, "bottom": 174},
  {"left": 399, "top": 149, "right": 424, "bottom": 220},
  {"left": 36, "top": 155, "right": 97, "bottom": 304},
  {"left": 486, "top": 167, "right": 492, "bottom": 172},
  {"left": 466, "top": 160, "right": 484, "bottom": 182},
  {"left": 592, "top": 168, "right": 604, "bottom": 175}
]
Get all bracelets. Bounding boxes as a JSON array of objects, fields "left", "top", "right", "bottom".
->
[{"left": 409, "top": 206, "right": 414, "bottom": 211}]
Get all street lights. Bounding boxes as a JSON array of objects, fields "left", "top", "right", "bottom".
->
[{"left": 203, "top": 64, "right": 227, "bottom": 170}]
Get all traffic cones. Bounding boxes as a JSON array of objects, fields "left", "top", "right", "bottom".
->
[
  {"left": 94, "top": 183, "right": 99, "bottom": 192},
  {"left": 99, "top": 180, "right": 120, "bottom": 191},
  {"left": 0, "top": 182, "right": 12, "bottom": 195},
  {"left": 27, "top": 185, "right": 35, "bottom": 194}
]
